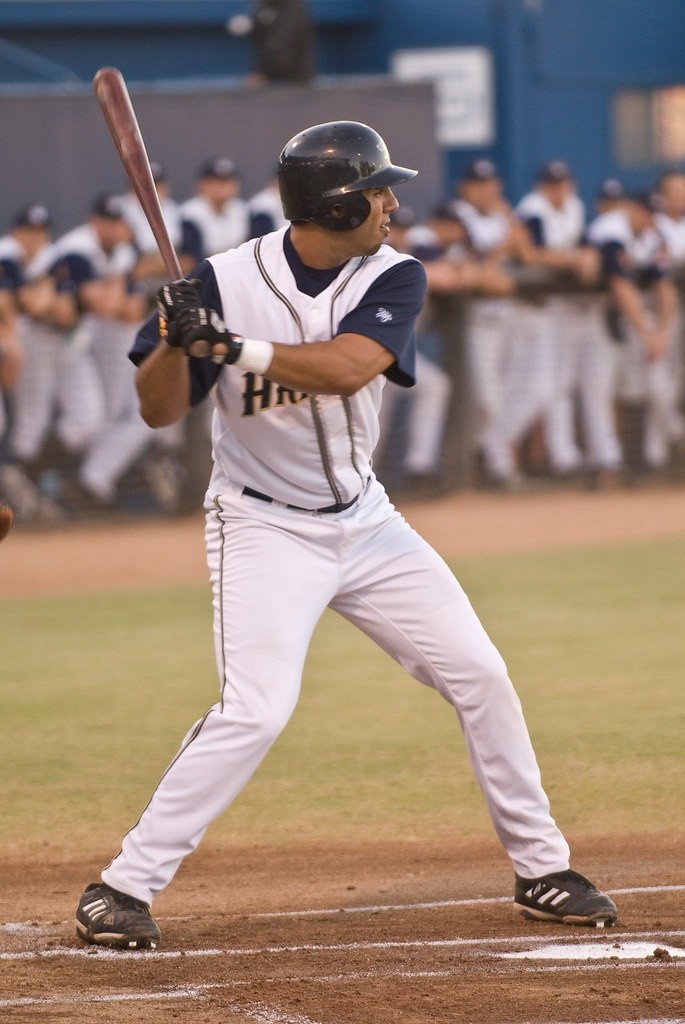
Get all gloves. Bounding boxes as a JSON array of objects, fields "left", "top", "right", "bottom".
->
[
  {"left": 156, "top": 278, "right": 203, "bottom": 347},
  {"left": 167, "top": 307, "right": 244, "bottom": 365}
]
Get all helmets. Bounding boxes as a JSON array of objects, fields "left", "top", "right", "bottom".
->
[{"left": 277, "top": 121, "right": 418, "bottom": 232}]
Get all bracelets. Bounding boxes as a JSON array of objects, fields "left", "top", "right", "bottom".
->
[{"left": 233, "top": 338, "right": 274, "bottom": 377}]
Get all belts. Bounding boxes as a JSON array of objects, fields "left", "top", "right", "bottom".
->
[{"left": 243, "top": 474, "right": 371, "bottom": 513}]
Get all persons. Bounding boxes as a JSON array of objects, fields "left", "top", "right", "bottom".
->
[
  {"left": 0, "top": 151, "right": 684, "bottom": 539},
  {"left": 76, "top": 118, "right": 620, "bottom": 952}
]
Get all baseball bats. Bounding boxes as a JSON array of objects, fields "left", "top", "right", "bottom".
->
[{"left": 92, "top": 68, "right": 210, "bottom": 358}]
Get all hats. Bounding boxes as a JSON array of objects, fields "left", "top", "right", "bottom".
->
[{"left": 16, "top": 145, "right": 625, "bottom": 232}]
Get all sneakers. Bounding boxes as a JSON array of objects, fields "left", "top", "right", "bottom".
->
[
  {"left": 513, "top": 868, "right": 618, "bottom": 928},
  {"left": 75, "top": 883, "right": 161, "bottom": 949}
]
